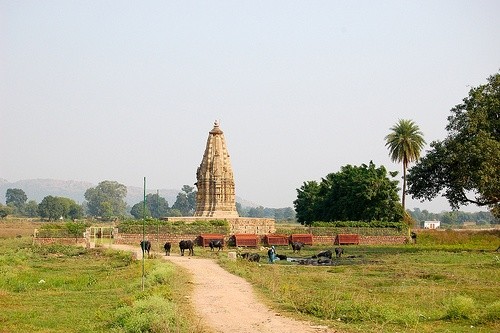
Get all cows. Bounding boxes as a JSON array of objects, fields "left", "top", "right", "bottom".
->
[
  {"left": 289, "top": 240, "right": 305, "bottom": 253},
  {"left": 164, "top": 242, "right": 171, "bottom": 255},
  {"left": 140, "top": 240, "right": 151, "bottom": 258},
  {"left": 209, "top": 240, "right": 224, "bottom": 251},
  {"left": 179, "top": 240, "right": 194, "bottom": 256},
  {"left": 275, "top": 254, "right": 287, "bottom": 261},
  {"left": 312, "top": 247, "right": 343, "bottom": 260},
  {"left": 237, "top": 252, "right": 260, "bottom": 262}
]
